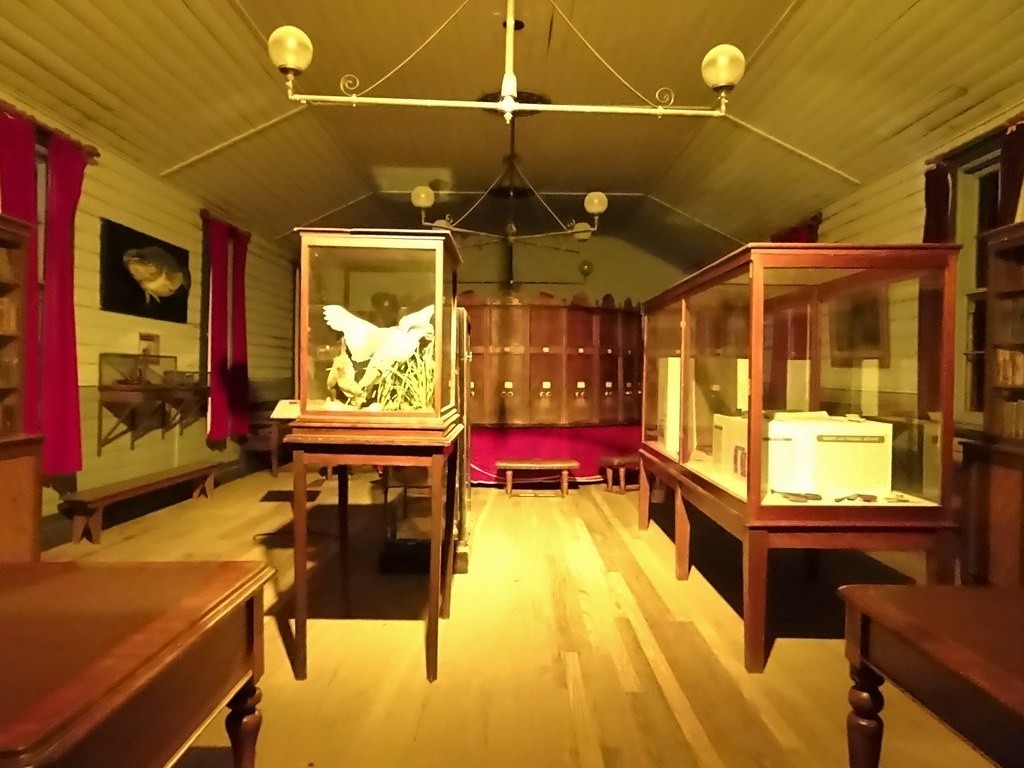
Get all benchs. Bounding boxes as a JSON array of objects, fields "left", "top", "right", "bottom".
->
[{"left": 59, "top": 461, "right": 225, "bottom": 544}]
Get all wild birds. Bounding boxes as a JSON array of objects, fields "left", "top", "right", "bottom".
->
[{"left": 323, "top": 304, "right": 435, "bottom": 384}]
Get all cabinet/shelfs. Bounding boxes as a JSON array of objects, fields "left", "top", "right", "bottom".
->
[
  {"left": 0, "top": 434, "right": 46, "bottom": 561},
  {"left": 975, "top": 221, "right": 1024, "bottom": 446},
  {"left": 957, "top": 441, "right": 1024, "bottom": 587},
  {"left": 0, "top": 213, "right": 35, "bottom": 441}
]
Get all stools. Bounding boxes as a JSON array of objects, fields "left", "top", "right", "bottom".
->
[
  {"left": 495, "top": 458, "right": 580, "bottom": 498},
  {"left": 599, "top": 454, "right": 640, "bottom": 493}
]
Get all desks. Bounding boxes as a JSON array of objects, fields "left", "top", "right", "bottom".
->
[
  {"left": 0, "top": 559, "right": 277, "bottom": 768},
  {"left": 836, "top": 585, "right": 1024, "bottom": 768},
  {"left": 282, "top": 422, "right": 465, "bottom": 683}
]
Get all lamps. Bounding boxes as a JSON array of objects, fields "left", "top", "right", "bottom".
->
[
  {"left": 410, "top": 186, "right": 435, "bottom": 226},
  {"left": 578, "top": 260, "right": 594, "bottom": 285},
  {"left": 268, "top": 25, "right": 314, "bottom": 101},
  {"left": 584, "top": 191, "right": 608, "bottom": 231},
  {"left": 433, "top": 220, "right": 451, "bottom": 232},
  {"left": 702, "top": 43, "right": 746, "bottom": 116},
  {"left": 573, "top": 223, "right": 592, "bottom": 254}
]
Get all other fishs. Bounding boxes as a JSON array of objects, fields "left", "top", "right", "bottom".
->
[{"left": 121, "top": 244, "right": 193, "bottom": 304}]
